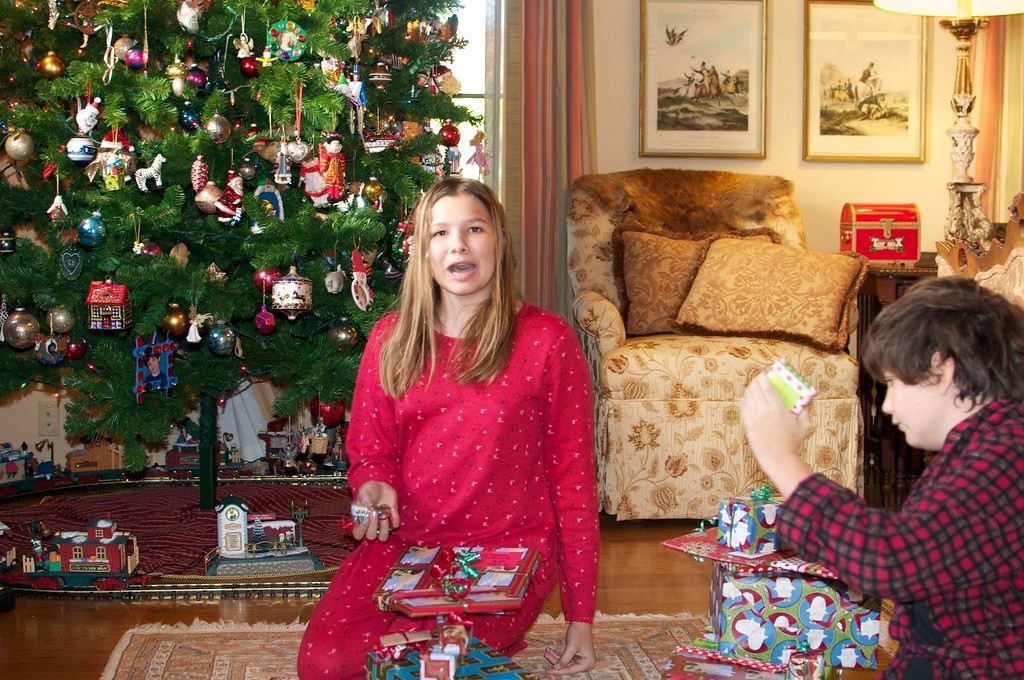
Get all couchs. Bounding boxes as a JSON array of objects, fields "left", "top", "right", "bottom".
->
[{"left": 566, "top": 167, "right": 863, "bottom": 520}]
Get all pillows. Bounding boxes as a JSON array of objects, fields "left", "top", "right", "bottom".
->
[
  {"left": 672, "top": 232, "right": 871, "bottom": 354},
  {"left": 613, "top": 224, "right": 782, "bottom": 338}
]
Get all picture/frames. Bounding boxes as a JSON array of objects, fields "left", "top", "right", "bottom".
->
[
  {"left": 639, "top": 0, "right": 767, "bottom": 160},
  {"left": 803, "top": 0, "right": 926, "bottom": 164}
]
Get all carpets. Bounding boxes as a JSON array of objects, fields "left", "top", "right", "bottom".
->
[{"left": 100, "top": 607, "right": 712, "bottom": 680}]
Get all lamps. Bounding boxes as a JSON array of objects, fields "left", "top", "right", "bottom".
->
[{"left": 874, "top": 1, "right": 1024, "bottom": 242}]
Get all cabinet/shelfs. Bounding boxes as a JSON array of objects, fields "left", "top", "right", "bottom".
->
[{"left": 855, "top": 253, "right": 937, "bottom": 506}]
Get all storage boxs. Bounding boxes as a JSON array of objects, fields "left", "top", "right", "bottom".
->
[
  {"left": 420, "top": 612, "right": 474, "bottom": 679},
  {"left": 840, "top": 202, "right": 922, "bottom": 264},
  {"left": 717, "top": 493, "right": 783, "bottom": 556},
  {"left": 709, "top": 560, "right": 881, "bottom": 670},
  {"left": 366, "top": 636, "right": 536, "bottom": 680},
  {"left": 767, "top": 357, "right": 818, "bottom": 416}
]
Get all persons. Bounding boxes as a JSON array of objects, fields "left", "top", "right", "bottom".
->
[
  {"left": 214, "top": 173, "right": 244, "bottom": 228},
  {"left": 274, "top": 141, "right": 292, "bottom": 184},
  {"left": 48, "top": 343, "right": 56, "bottom": 355},
  {"left": 738, "top": 277, "right": 1024, "bottom": 680},
  {"left": 298, "top": 179, "right": 600, "bottom": 680},
  {"left": 466, "top": 132, "right": 488, "bottom": 183},
  {"left": 320, "top": 135, "right": 345, "bottom": 202},
  {"left": 349, "top": 65, "right": 366, "bottom": 111},
  {"left": 143, "top": 353, "right": 167, "bottom": 384}
]
